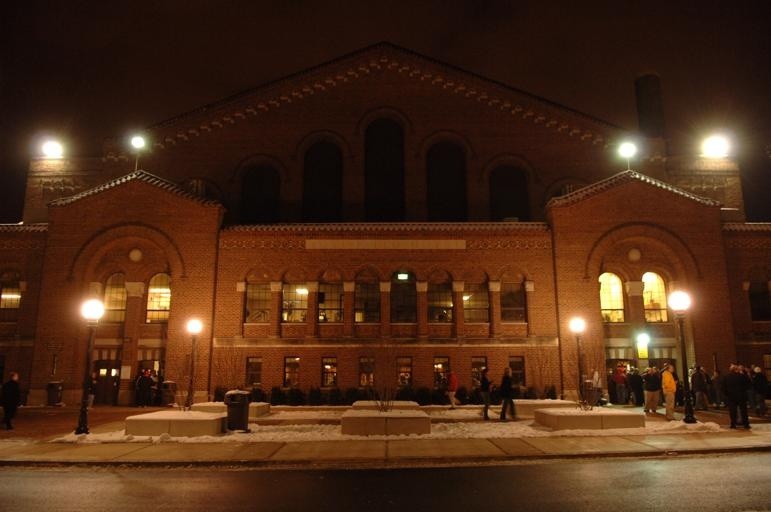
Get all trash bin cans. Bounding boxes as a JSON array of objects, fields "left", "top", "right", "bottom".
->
[
  {"left": 48, "top": 381, "right": 63, "bottom": 405},
  {"left": 584, "top": 380, "right": 599, "bottom": 406},
  {"left": 224, "top": 390, "right": 250, "bottom": 430}
]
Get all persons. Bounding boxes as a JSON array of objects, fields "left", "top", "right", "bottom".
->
[
  {"left": 442, "top": 368, "right": 462, "bottom": 410},
  {"left": 135, "top": 369, "right": 159, "bottom": 408},
  {"left": 88, "top": 370, "right": 98, "bottom": 410},
  {"left": 0, "top": 371, "right": 23, "bottom": 430},
  {"left": 498, "top": 367, "right": 521, "bottom": 421},
  {"left": 479, "top": 368, "right": 496, "bottom": 420},
  {"left": 610, "top": 361, "right": 771, "bottom": 429}
]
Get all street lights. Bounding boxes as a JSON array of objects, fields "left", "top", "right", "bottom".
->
[
  {"left": 186, "top": 316, "right": 202, "bottom": 412},
  {"left": 569, "top": 316, "right": 586, "bottom": 401},
  {"left": 71, "top": 299, "right": 105, "bottom": 436},
  {"left": 668, "top": 291, "right": 698, "bottom": 423}
]
